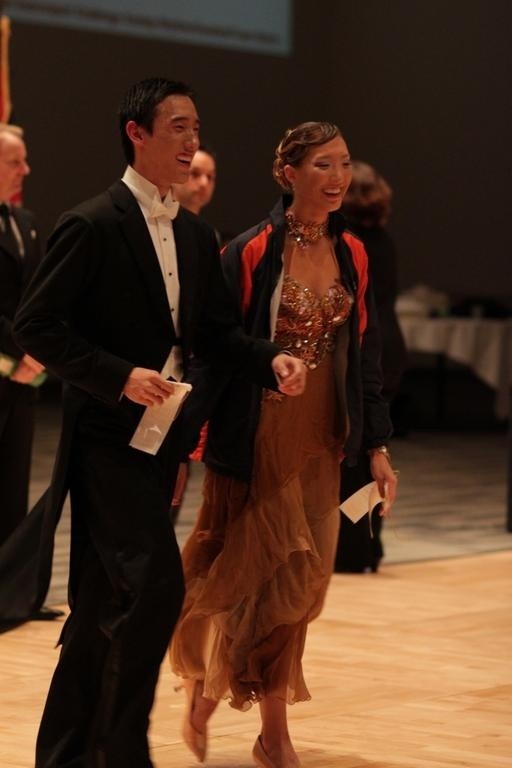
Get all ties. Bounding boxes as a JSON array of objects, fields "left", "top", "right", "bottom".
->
[{"left": 0, "top": 202, "right": 21, "bottom": 263}]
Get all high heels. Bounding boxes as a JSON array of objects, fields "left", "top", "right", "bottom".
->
[
  {"left": 336, "top": 541, "right": 383, "bottom": 573},
  {"left": 252, "top": 736, "right": 276, "bottom": 767},
  {"left": 173, "top": 679, "right": 207, "bottom": 762}
]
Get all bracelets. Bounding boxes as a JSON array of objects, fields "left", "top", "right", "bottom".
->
[{"left": 366, "top": 446, "right": 394, "bottom": 462}]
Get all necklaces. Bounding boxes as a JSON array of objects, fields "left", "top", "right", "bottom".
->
[{"left": 283, "top": 210, "right": 333, "bottom": 252}]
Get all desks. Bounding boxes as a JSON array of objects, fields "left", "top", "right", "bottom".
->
[{"left": 396, "top": 315, "right": 512, "bottom": 423}]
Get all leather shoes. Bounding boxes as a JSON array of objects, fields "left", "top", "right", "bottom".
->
[{"left": 36, "top": 604, "right": 62, "bottom": 619}]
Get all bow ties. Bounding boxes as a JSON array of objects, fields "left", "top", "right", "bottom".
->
[{"left": 116, "top": 178, "right": 180, "bottom": 223}]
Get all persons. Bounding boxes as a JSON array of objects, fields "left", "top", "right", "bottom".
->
[
  {"left": 320, "top": 160, "right": 410, "bottom": 578},
  {"left": 10, "top": 74, "right": 311, "bottom": 767},
  {"left": 163, "top": 123, "right": 400, "bottom": 768},
  {"left": 0, "top": 125, "right": 67, "bottom": 638},
  {"left": 172, "top": 147, "right": 225, "bottom": 253}
]
